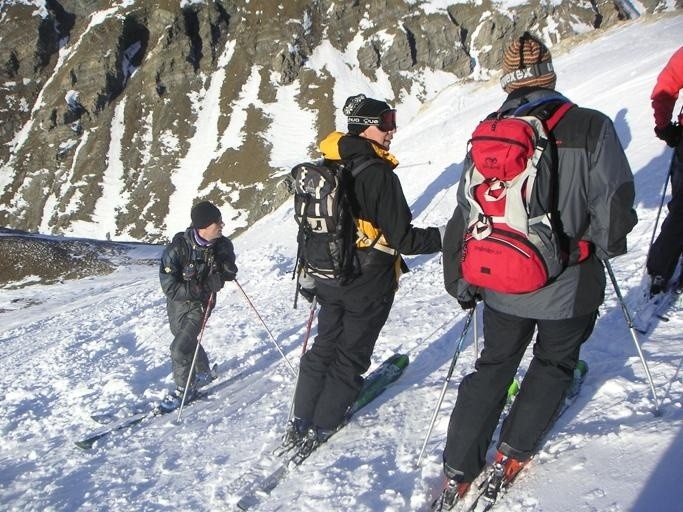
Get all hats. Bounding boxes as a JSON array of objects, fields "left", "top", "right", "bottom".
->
[
  {"left": 342, "top": 94, "right": 389, "bottom": 133},
  {"left": 500, "top": 31, "right": 556, "bottom": 94},
  {"left": 191, "top": 200, "right": 220, "bottom": 230}
]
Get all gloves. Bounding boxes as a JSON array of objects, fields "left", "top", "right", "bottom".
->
[
  {"left": 654, "top": 122, "right": 681, "bottom": 147},
  {"left": 215, "top": 236, "right": 234, "bottom": 260},
  {"left": 202, "top": 271, "right": 225, "bottom": 292}
]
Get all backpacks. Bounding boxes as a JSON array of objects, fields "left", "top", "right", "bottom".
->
[
  {"left": 456, "top": 99, "right": 593, "bottom": 294},
  {"left": 283, "top": 161, "right": 374, "bottom": 287}
]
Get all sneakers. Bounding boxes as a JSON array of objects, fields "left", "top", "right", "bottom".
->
[
  {"left": 495, "top": 453, "right": 526, "bottom": 481},
  {"left": 293, "top": 417, "right": 313, "bottom": 435},
  {"left": 643, "top": 273, "right": 667, "bottom": 295},
  {"left": 308, "top": 418, "right": 345, "bottom": 442},
  {"left": 159, "top": 386, "right": 198, "bottom": 412},
  {"left": 194, "top": 370, "right": 212, "bottom": 385},
  {"left": 445, "top": 476, "right": 467, "bottom": 496}
]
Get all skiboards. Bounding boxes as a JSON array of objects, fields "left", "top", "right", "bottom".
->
[
  {"left": 227, "top": 353, "right": 409, "bottom": 507},
  {"left": 73, "top": 364, "right": 222, "bottom": 450},
  {"left": 429, "top": 358, "right": 588, "bottom": 512},
  {"left": 626, "top": 279, "right": 683, "bottom": 336}
]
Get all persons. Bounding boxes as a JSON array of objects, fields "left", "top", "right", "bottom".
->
[
  {"left": 438, "top": 28, "right": 638, "bottom": 507},
  {"left": 159, "top": 201, "right": 238, "bottom": 413},
  {"left": 282, "top": 94, "right": 449, "bottom": 453},
  {"left": 645, "top": 46, "right": 682, "bottom": 299}
]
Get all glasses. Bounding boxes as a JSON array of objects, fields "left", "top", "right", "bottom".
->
[{"left": 375, "top": 109, "right": 397, "bottom": 132}]
